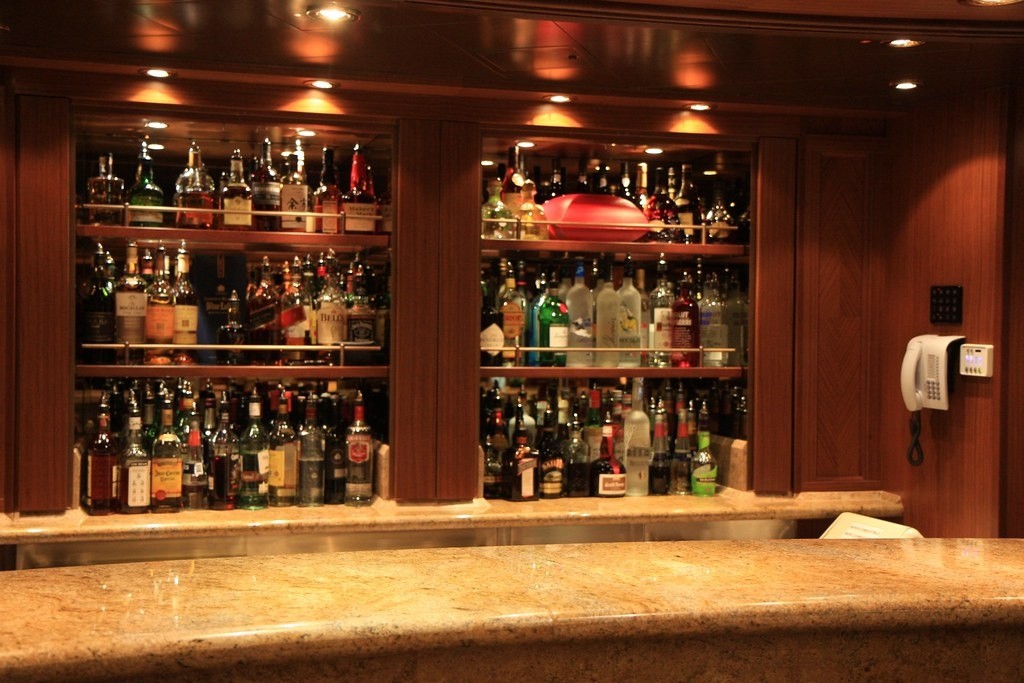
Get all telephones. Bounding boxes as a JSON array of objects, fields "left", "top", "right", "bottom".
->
[{"left": 899, "top": 334, "right": 966, "bottom": 411}]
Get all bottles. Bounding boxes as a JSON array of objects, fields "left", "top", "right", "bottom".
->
[
  {"left": 480, "top": 377, "right": 746, "bottom": 502},
  {"left": 479, "top": 250, "right": 746, "bottom": 368},
  {"left": 202, "top": 249, "right": 391, "bottom": 366},
  {"left": 126, "top": 143, "right": 164, "bottom": 228},
  {"left": 173, "top": 138, "right": 392, "bottom": 234},
  {"left": 75, "top": 238, "right": 199, "bottom": 365},
  {"left": 76, "top": 152, "right": 125, "bottom": 224},
  {"left": 481, "top": 145, "right": 750, "bottom": 243},
  {"left": 72, "top": 377, "right": 390, "bottom": 517}
]
